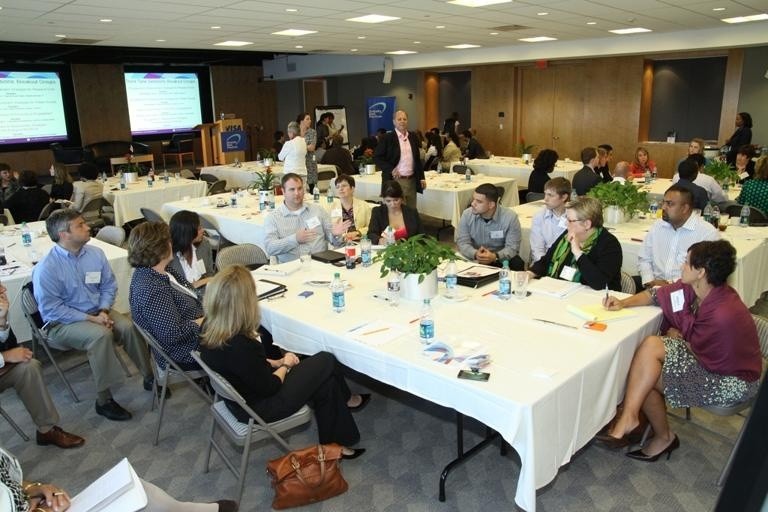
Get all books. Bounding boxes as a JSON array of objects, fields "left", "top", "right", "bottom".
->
[
  {"left": 445, "top": 264, "right": 501, "bottom": 289},
  {"left": 252, "top": 276, "right": 289, "bottom": 305},
  {"left": 312, "top": 250, "right": 348, "bottom": 264}
]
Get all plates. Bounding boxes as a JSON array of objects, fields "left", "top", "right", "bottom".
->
[
  {"left": 440, "top": 291, "right": 469, "bottom": 301},
  {"left": 306, "top": 277, "right": 332, "bottom": 286}
]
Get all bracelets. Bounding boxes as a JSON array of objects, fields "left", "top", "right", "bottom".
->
[
  {"left": 35, "top": 507, "right": 46, "bottom": 512},
  {"left": 22, "top": 479, "right": 42, "bottom": 491},
  {"left": 495, "top": 251, "right": 500, "bottom": 264}
]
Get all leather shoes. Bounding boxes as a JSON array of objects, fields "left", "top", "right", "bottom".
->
[
  {"left": 349, "top": 393, "right": 371, "bottom": 411},
  {"left": 144, "top": 379, "right": 171, "bottom": 398},
  {"left": 595, "top": 425, "right": 641, "bottom": 450},
  {"left": 343, "top": 449, "right": 365, "bottom": 459},
  {"left": 37, "top": 426, "right": 83, "bottom": 448},
  {"left": 95, "top": 398, "right": 130, "bottom": 420}
]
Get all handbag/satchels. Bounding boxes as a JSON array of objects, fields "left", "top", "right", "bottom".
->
[{"left": 266, "top": 445, "right": 348, "bottom": 508}]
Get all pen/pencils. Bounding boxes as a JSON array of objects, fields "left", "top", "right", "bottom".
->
[
  {"left": 409, "top": 314, "right": 431, "bottom": 325},
  {"left": 605, "top": 285, "right": 609, "bottom": 299},
  {"left": 360, "top": 327, "right": 388, "bottom": 338}
]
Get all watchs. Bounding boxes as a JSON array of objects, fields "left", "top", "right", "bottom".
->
[{"left": 279, "top": 362, "right": 292, "bottom": 374}]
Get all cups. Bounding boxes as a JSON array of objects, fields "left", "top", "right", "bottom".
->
[
  {"left": 717, "top": 211, "right": 731, "bottom": 232},
  {"left": 513, "top": 271, "right": 529, "bottom": 300},
  {"left": 175, "top": 173, "right": 180, "bottom": 179},
  {"left": 299, "top": 243, "right": 313, "bottom": 270},
  {"left": 184, "top": 195, "right": 190, "bottom": 202}
]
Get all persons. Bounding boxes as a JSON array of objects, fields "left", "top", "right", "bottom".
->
[
  {"left": 1, "top": 168, "right": 51, "bottom": 223},
  {"left": 328, "top": 174, "right": 375, "bottom": 249},
  {"left": 0, "top": 279, "right": 87, "bottom": 452},
  {"left": 269, "top": 112, "right": 353, "bottom": 194},
  {"left": 197, "top": 262, "right": 374, "bottom": 461},
  {"left": 636, "top": 184, "right": 724, "bottom": 338},
  {"left": 48, "top": 159, "right": 74, "bottom": 212},
  {"left": 452, "top": 181, "right": 525, "bottom": 269},
  {"left": 725, "top": 113, "right": 754, "bottom": 164},
  {"left": 594, "top": 235, "right": 764, "bottom": 465},
  {"left": 259, "top": 172, "right": 353, "bottom": 264},
  {"left": 375, "top": 110, "right": 425, "bottom": 209},
  {"left": 673, "top": 139, "right": 767, "bottom": 219},
  {"left": 365, "top": 179, "right": 426, "bottom": 247},
  {"left": 0, "top": 162, "right": 18, "bottom": 213},
  {"left": 29, "top": 206, "right": 172, "bottom": 423},
  {"left": 443, "top": 112, "right": 460, "bottom": 134},
  {"left": 522, "top": 150, "right": 560, "bottom": 204},
  {"left": 353, "top": 128, "right": 488, "bottom": 173},
  {"left": 68, "top": 163, "right": 105, "bottom": 223},
  {"left": 528, "top": 175, "right": 571, "bottom": 277},
  {"left": 0, "top": 445, "right": 242, "bottom": 512},
  {"left": 515, "top": 194, "right": 624, "bottom": 292},
  {"left": 573, "top": 145, "right": 613, "bottom": 195},
  {"left": 126, "top": 218, "right": 213, "bottom": 395},
  {"left": 626, "top": 146, "right": 657, "bottom": 178},
  {"left": 166, "top": 208, "right": 218, "bottom": 289}
]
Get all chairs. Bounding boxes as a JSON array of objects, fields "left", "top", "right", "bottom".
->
[
  {"left": 18, "top": 279, "right": 131, "bottom": 405},
  {"left": 189, "top": 349, "right": 315, "bottom": 509},
  {"left": 2, "top": 139, "right": 768, "bottom": 310},
  {"left": 639, "top": 312, "right": 767, "bottom": 485},
  {"left": 132, "top": 318, "right": 213, "bottom": 448}
]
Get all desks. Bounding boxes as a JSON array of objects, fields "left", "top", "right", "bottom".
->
[
  {"left": 1, "top": 221, "right": 132, "bottom": 349},
  {"left": 246, "top": 241, "right": 664, "bottom": 509}
]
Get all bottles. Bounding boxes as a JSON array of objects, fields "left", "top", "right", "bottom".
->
[
  {"left": 19, "top": 221, "right": 31, "bottom": 246},
  {"left": 703, "top": 200, "right": 720, "bottom": 228},
  {"left": 201, "top": 185, "right": 334, "bottom": 212},
  {"left": 649, "top": 195, "right": 659, "bottom": 218},
  {"left": 420, "top": 298, "right": 434, "bottom": 343},
  {"left": 569, "top": 187, "right": 578, "bottom": 204},
  {"left": 344, "top": 235, "right": 356, "bottom": 269},
  {"left": 644, "top": 169, "right": 651, "bottom": 185},
  {"left": 444, "top": 258, "right": 458, "bottom": 295},
  {"left": 465, "top": 166, "right": 471, "bottom": 183},
  {"left": 0, "top": 247, "right": 7, "bottom": 265},
  {"left": 739, "top": 202, "right": 751, "bottom": 227},
  {"left": 332, "top": 273, "right": 346, "bottom": 313},
  {"left": 385, "top": 226, "right": 396, "bottom": 250},
  {"left": 388, "top": 266, "right": 401, "bottom": 306},
  {"left": 359, "top": 232, "right": 372, "bottom": 268},
  {"left": 256, "top": 151, "right": 261, "bottom": 161},
  {"left": 498, "top": 261, "right": 512, "bottom": 300},
  {"left": 437, "top": 161, "right": 442, "bottom": 175},
  {"left": 220, "top": 112, "right": 225, "bottom": 120},
  {"left": 722, "top": 176, "right": 729, "bottom": 192},
  {"left": 31, "top": 248, "right": 40, "bottom": 267},
  {"left": 651, "top": 165, "right": 657, "bottom": 183},
  {"left": 98, "top": 167, "right": 170, "bottom": 192}
]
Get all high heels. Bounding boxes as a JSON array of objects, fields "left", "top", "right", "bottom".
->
[{"left": 626, "top": 433, "right": 679, "bottom": 463}]
